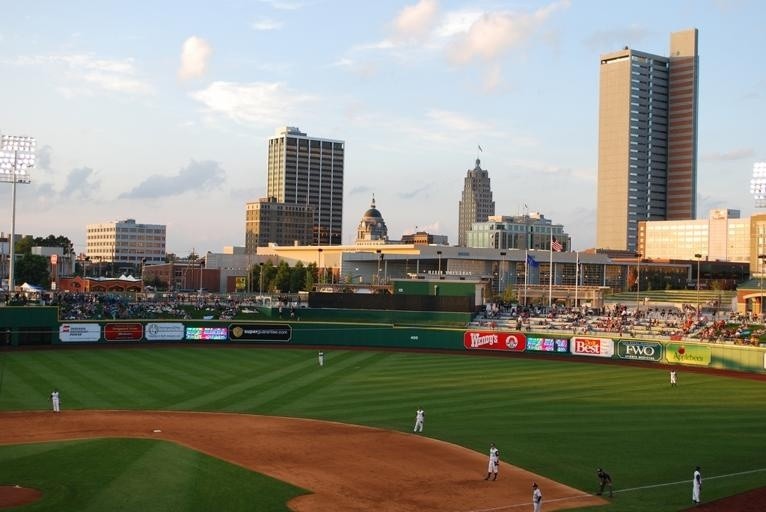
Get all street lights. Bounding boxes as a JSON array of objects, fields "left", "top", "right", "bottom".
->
[
  {"left": 501, "top": 252, "right": 506, "bottom": 281},
  {"left": 437, "top": 251, "right": 441, "bottom": 272},
  {"left": 0, "top": 136, "right": 36, "bottom": 291},
  {"left": 758, "top": 255, "right": 766, "bottom": 314},
  {"left": 377, "top": 249, "right": 381, "bottom": 285},
  {"left": 82, "top": 256, "right": 90, "bottom": 292},
  {"left": 260, "top": 262, "right": 264, "bottom": 294},
  {"left": 318, "top": 248, "right": 322, "bottom": 278},
  {"left": 695, "top": 254, "right": 701, "bottom": 315},
  {"left": 141, "top": 257, "right": 147, "bottom": 293},
  {"left": 635, "top": 254, "right": 642, "bottom": 309}
]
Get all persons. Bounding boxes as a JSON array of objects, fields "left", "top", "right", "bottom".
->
[
  {"left": 318, "top": 349, "right": 324, "bottom": 368},
  {"left": 48, "top": 388, "right": 64, "bottom": 412},
  {"left": 596, "top": 468, "right": 613, "bottom": 497},
  {"left": 488, "top": 296, "right": 761, "bottom": 347},
  {"left": 412, "top": 405, "right": 426, "bottom": 433},
  {"left": 670, "top": 368, "right": 679, "bottom": 389},
  {"left": 531, "top": 480, "right": 543, "bottom": 512},
  {"left": 3, "top": 285, "right": 241, "bottom": 320},
  {"left": 278, "top": 295, "right": 304, "bottom": 321},
  {"left": 484, "top": 441, "right": 501, "bottom": 482},
  {"left": 691, "top": 466, "right": 702, "bottom": 504}
]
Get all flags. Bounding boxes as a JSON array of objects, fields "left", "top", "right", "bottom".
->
[
  {"left": 552, "top": 234, "right": 563, "bottom": 252},
  {"left": 527, "top": 254, "right": 539, "bottom": 267},
  {"left": 578, "top": 258, "right": 581, "bottom": 267}
]
